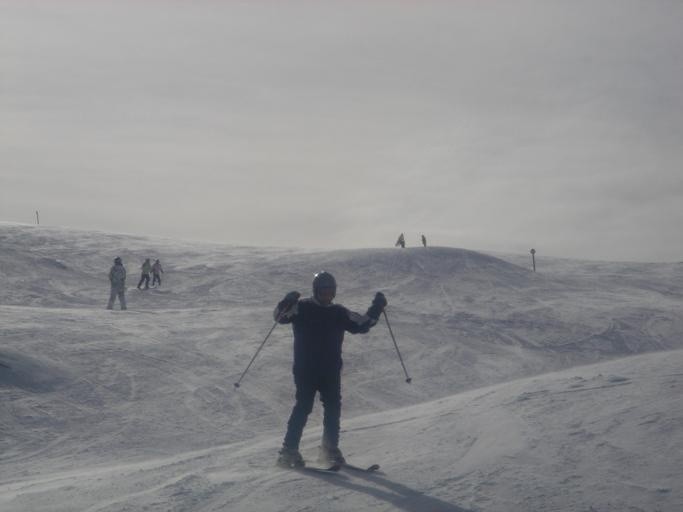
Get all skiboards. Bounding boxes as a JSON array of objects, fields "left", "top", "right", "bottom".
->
[
  {"left": 131, "top": 285, "right": 163, "bottom": 289},
  {"left": 247, "top": 456, "right": 380, "bottom": 474}
]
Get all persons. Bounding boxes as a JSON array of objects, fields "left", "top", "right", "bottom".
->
[
  {"left": 421, "top": 234, "right": 426, "bottom": 246},
  {"left": 134, "top": 257, "right": 152, "bottom": 288},
  {"left": 150, "top": 259, "right": 162, "bottom": 286},
  {"left": 273, "top": 270, "right": 387, "bottom": 465},
  {"left": 104, "top": 256, "right": 127, "bottom": 311},
  {"left": 396, "top": 231, "right": 405, "bottom": 249}
]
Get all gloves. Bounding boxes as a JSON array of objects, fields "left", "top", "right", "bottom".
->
[
  {"left": 276, "top": 290, "right": 301, "bottom": 313},
  {"left": 366, "top": 291, "right": 388, "bottom": 321}
]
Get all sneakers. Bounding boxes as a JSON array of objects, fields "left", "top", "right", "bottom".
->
[
  {"left": 275, "top": 446, "right": 306, "bottom": 469},
  {"left": 315, "top": 444, "right": 346, "bottom": 464}
]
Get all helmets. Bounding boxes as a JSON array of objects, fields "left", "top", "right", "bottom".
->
[{"left": 312, "top": 270, "right": 337, "bottom": 299}]
